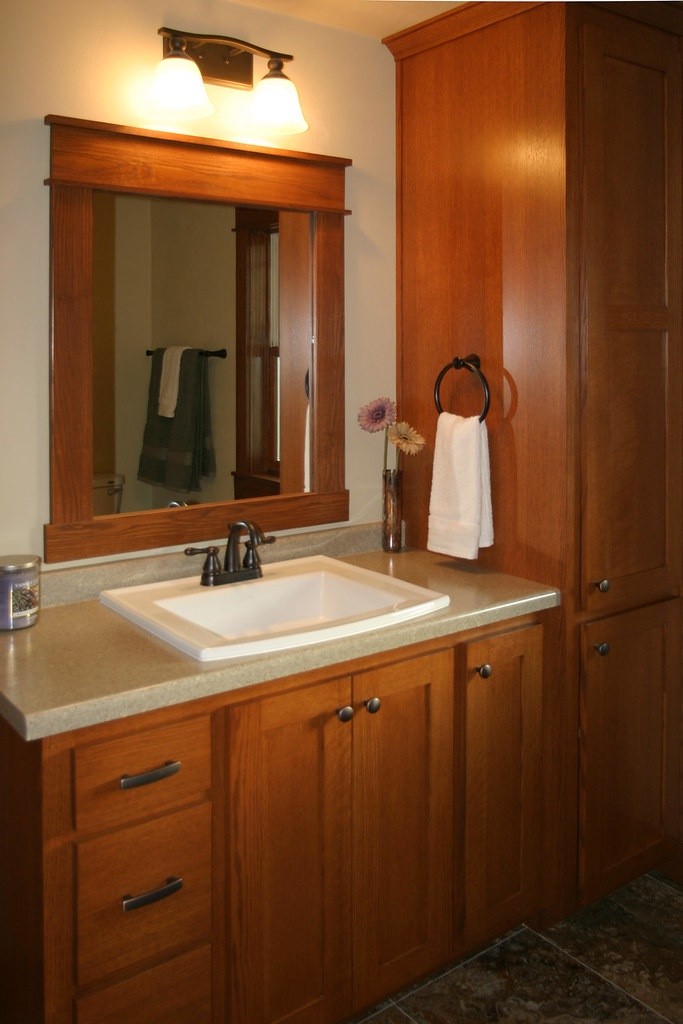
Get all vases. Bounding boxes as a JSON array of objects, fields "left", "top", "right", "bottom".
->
[{"left": 382, "top": 470, "right": 403, "bottom": 552}]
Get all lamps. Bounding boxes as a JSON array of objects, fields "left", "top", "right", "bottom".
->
[{"left": 152, "top": 27, "right": 309, "bottom": 135}]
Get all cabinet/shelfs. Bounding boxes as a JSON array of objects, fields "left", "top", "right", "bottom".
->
[
  {"left": 0, "top": 693, "right": 220, "bottom": 1024},
  {"left": 220, "top": 634, "right": 453, "bottom": 1024},
  {"left": 382, "top": 1, "right": 683, "bottom": 907},
  {"left": 453, "top": 606, "right": 564, "bottom": 955}
]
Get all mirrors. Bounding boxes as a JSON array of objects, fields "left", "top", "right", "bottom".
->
[{"left": 43, "top": 114, "right": 352, "bottom": 564}]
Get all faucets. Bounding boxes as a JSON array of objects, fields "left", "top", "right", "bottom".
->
[
  {"left": 166, "top": 500, "right": 188, "bottom": 507},
  {"left": 184, "top": 522, "right": 276, "bottom": 589}
]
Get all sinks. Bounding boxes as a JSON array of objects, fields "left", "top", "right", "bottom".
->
[{"left": 97, "top": 554, "right": 455, "bottom": 661}]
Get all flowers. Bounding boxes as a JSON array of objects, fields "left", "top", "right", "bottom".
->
[{"left": 359, "top": 397, "right": 427, "bottom": 472}]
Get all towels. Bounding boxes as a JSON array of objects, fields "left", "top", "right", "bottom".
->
[
  {"left": 133, "top": 345, "right": 217, "bottom": 491},
  {"left": 426, "top": 411, "right": 494, "bottom": 560}
]
